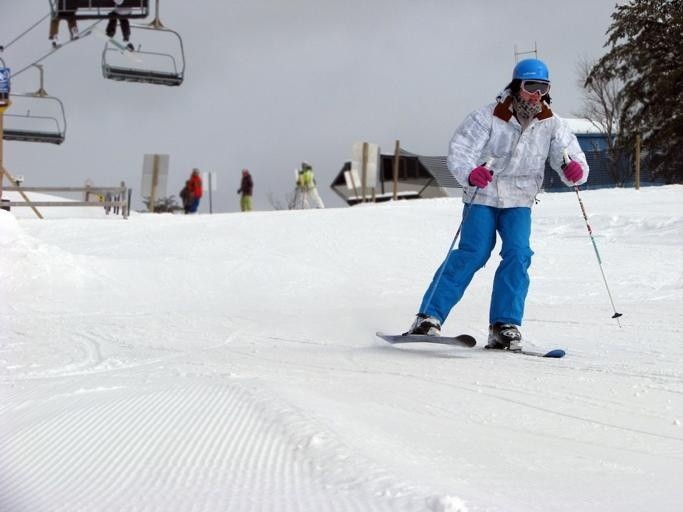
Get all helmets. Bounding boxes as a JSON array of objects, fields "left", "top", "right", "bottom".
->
[{"left": 513, "top": 59, "right": 548, "bottom": 81}]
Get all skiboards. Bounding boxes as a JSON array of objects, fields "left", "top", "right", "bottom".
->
[{"left": 375, "top": 329, "right": 565, "bottom": 358}]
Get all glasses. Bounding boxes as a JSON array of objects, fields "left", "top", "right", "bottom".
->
[{"left": 521, "top": 80, "right": 549, "bottom": 96}]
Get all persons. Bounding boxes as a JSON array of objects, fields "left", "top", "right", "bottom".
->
[
  {"left": 47, "top": 0, "right": 79, "bottom": 48},
  {"left": 187, "top": 169, "right": 204, "bottom": 213},
  {"left": 403, "top": 58, "right": 591, "bottom": 352},
  {"left": 104, "top": 8, "right": 130, "bottom": 44},
  {"left": 235, "top": 169, "right": 253, "bottom": 212},
  {"left": 293, "top": 161, "right": 325, "bottom": 209},
  {"left": 179, "top": 181, "right": 188, "bottom": 214}
]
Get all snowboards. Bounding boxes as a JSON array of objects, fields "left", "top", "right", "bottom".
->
[{"left": 48, "top": 26, "right": 136, "bottom": 55}]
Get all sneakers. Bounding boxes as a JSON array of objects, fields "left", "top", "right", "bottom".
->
[
  {"left": 410, "top": 316, "right": 440, "bottom": 335},
  {"left": 488, "top": 322, "right": 521, "bottom": 345}
]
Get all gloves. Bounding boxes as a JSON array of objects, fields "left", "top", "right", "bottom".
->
[
  {"left": 564, "top": 160, "right": 583, "bottom": 182},
  {"left": 470, "top": 167, "right": 492, "bottom": 188}
]
